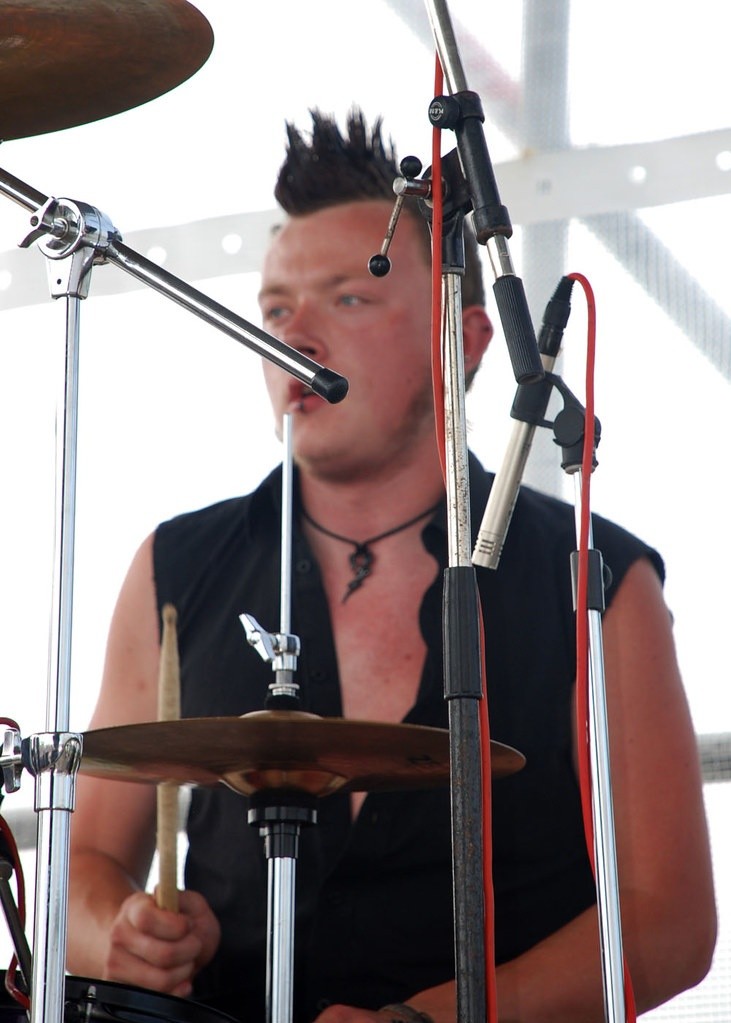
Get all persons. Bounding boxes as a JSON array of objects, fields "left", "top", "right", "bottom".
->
[{"left": 64, "top": 108, "right": 718, "bottom": 1023}]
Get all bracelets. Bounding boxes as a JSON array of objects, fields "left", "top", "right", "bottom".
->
[{"left": 380, "top": 1001, "right": 434, "bottom": 1023}]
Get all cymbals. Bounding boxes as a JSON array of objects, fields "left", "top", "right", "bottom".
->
[
  {"left": 73, "top": 706, "right": 528, "bottom": 805},
  {"left": 0, "top": 0, "right": 216, "bottom": 143}
]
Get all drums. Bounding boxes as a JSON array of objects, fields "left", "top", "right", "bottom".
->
[{"left": 0, "top": 968, "right": 243, "bottom": 1023}]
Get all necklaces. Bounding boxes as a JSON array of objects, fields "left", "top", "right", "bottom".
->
[{"left": 300, "top": 491, "right": 442, "bottom": 607}]
